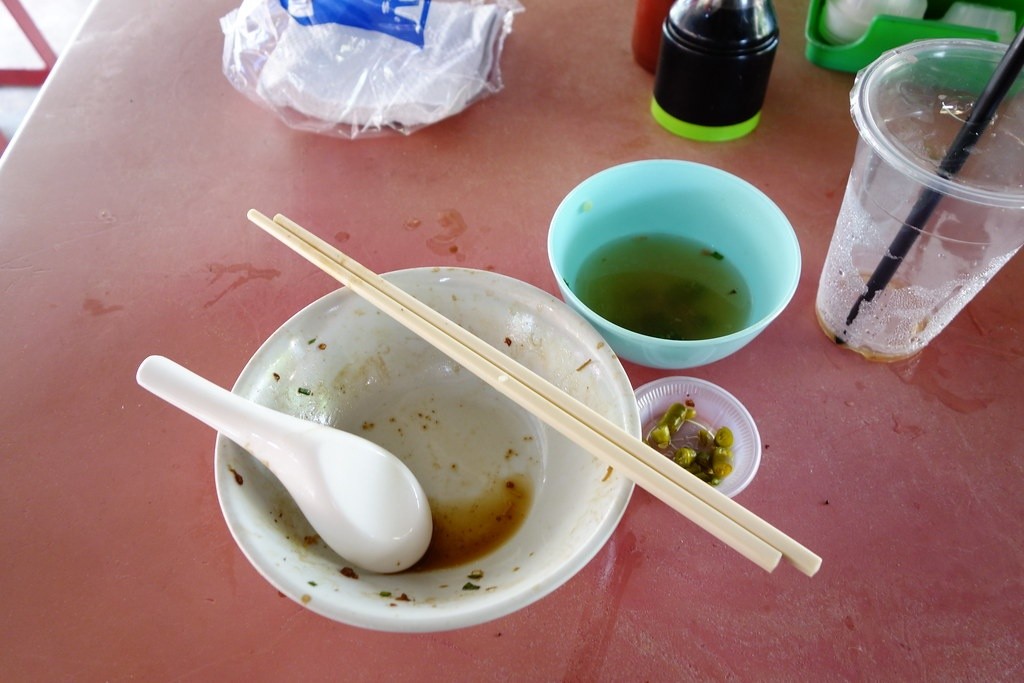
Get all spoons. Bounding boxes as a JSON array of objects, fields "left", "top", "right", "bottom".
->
[{"left": 136, "top": 356, "right": 431, "bottom": 572}]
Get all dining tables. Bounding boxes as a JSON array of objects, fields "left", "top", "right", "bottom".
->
[{"left": 0, "top": 0, "right": 1024, "bottom": 683}]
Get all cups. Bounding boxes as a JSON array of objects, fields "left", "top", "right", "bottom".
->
[
  {"left": 632, "top": 0, "right": 676, "bottom": 75},
  {"left": 813, "top": 38, "right": 1024, "bottom": 361}
]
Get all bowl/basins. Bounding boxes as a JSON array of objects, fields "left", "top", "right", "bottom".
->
[
  {"left": 215, "top": 267, "right": 641, "bottom": 633},
  {"left": 550, "top": 157, "right": 802, "bottom": 367},
  {"left": 635, "top": 377, "right": 762, "bottom": 499}
]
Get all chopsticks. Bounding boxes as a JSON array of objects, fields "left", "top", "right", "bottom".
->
[{"left": 243, "top": 206, "right": 822, "bottom": 580}]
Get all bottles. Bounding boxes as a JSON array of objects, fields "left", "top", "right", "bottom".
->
[{"left": 651, "top": 0, "right": 780, "bottom": 142}]
